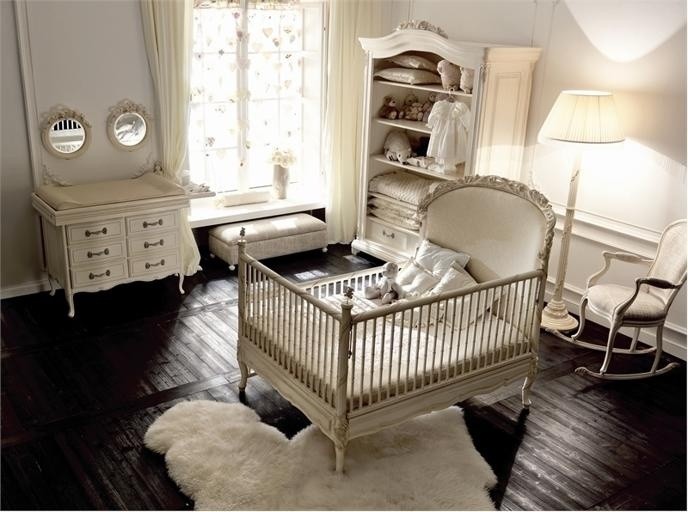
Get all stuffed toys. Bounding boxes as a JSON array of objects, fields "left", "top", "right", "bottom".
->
[
  {"left": 379, "top": 92, "right": 453, "bottom": 120},
  {"left": 363, "top": 262, "right": 402, "bottom": 303},
  {"left": 437, "top": 60, "right": 474, "bottom": 92},
  {"left": 383, "top": 129, "right": 412, "bottom": 164}
]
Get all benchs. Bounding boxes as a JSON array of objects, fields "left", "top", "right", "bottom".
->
[{"left": 205, "top": 213, "right": 329, "bottom": 271}]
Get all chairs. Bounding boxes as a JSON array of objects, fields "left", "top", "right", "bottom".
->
[{"left": 552, "top": 219, "right": 688, "bottom": 380}]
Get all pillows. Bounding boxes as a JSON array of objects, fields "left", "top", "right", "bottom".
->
[{"left": 395, "top": 239, "right": 506, "bottom": 331}]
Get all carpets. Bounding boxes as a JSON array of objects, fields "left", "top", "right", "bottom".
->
[{"left": 143, "top": 399, "right": 502, "bottom": 510}]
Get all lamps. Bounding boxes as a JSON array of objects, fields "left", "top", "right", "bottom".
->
[{"left": 535, "top": 88, "right": 627, "bottom": 335}]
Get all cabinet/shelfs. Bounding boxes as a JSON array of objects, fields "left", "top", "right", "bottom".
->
[
  {"left": 352, "top": 29, "right": 545, "bottom": 269},
  {"left": 30, "top": 170, "right": 190, "bottom": 315}
]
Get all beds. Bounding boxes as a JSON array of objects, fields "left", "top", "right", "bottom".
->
[{"left": 237, "top": 170, "right": 556, "bottom": 475}]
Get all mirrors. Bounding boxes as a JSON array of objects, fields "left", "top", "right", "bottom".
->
[{"left": 37, "top": 98, "right": 153, "bottom": 159}]
